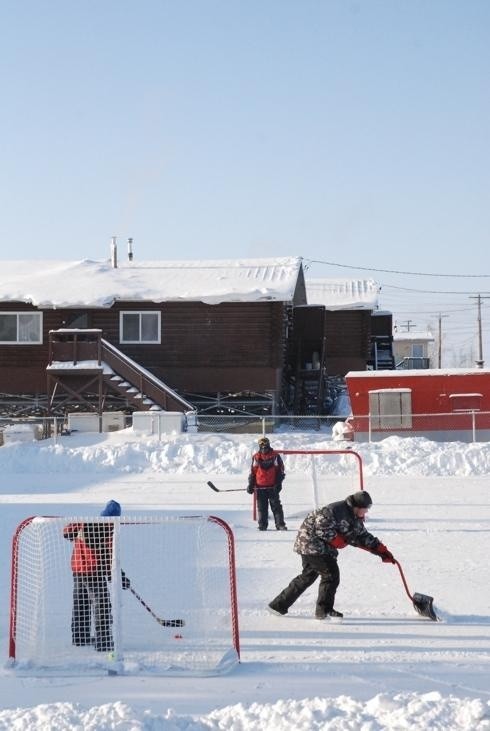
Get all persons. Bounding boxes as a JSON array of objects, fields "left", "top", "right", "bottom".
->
[
  {"left": 266, "top": 489, "right": 399, "bottom": 619},
  {"left": 247, "top": 437, "right": 288, "bottom": 532},
  {"left": 60, "top": 500, "right": 131, "bottom": 653}
]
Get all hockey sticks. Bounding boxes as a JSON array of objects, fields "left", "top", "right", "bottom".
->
[{"left": 207, "top": 481, "right": 274, "bottom": 492}]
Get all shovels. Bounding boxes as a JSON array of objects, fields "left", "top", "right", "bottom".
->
[
  {"left": 395, "top": 559, "right": 438, "bottom": 621},
  {"left": 208, "top": 480, "right": 246, "bottom": 492}
]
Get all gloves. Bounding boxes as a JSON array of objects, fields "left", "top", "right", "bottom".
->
[
  {"left": 122, "top": 572, "right": 130, "bottom": 590},
  {"left": 375, "top": 545, "right": 395, "bottom": 564},
  {"left": 247, "top": 485, "right": 253, "bottom": 494},
  {"left": 331, "top": 535, "right": 347, "bottom": 548},
  {"left": 274, "top": 483, "right": 282, "bottom": 493}
]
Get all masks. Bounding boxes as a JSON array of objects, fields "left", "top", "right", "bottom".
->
[{"left": 261, "top": 448, "right": 269, "bottom": 453}]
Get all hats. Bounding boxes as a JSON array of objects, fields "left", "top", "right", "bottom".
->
[
  {"left": 259, "top": 438, "right": 269, "bottom": 447},
  {"left": 100, "top": 501, "right": 121, "bottom": 515},
  {"left": 352, "top": 491, "right": 371, "bottom": 508}
]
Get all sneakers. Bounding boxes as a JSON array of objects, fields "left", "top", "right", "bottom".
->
[
  {"left": 268, "top": 602, "right": 287, "bottom": 615},
  {"left": 315, "top": 609, "right": 343, "bottom": 621},
  {"left": 258, "top": 526, "right": 267, "bottom": 530},
  {"left": 276, "top": 525, "right": 287, "bottom": 530},
  {"left": 72, "top": 637, "right": 115, "bottom": 652}
]
